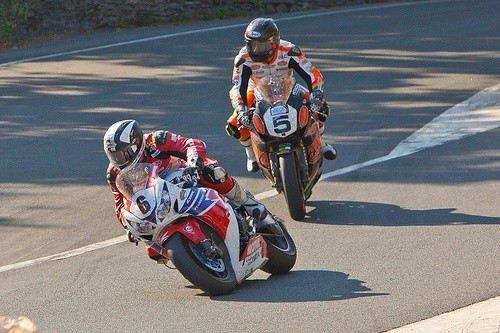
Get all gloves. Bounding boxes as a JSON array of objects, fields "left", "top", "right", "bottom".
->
[
  {"left": 236, "top": 104, "right": 250, "bottom": 126},
  {"left": 310, "top": 90, "right": 323, "bottom": 114},
  {"left": 181, "top": 164, "right": 202, "bottom": 183},
  {"left": 124, "top": 226, "right": 141, "bottom": 243}
]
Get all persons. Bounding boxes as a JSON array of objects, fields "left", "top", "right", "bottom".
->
[
  {"left": 224, "top": 17, "right": 338, "bottom": 174},
  {"left": 103, "top": 119, "right": 267, "bottom": 267}
]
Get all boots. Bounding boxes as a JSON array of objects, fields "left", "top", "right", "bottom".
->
[
  {"left": 318, "top": 121, "right": 337, "bottom": 160},
  {"left": 223, "top": 177, "right": 267, "bottom": 221},
  {"left": 239, "top": 135, "right": 259, "bottom": 173}
]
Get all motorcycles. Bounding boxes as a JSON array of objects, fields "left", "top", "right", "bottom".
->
[
  {"left": 115, "top": 161, "right": 298, "bottom": 297},
  {"left": 236, "top": 68, "right": 324, "bottom": 223}
]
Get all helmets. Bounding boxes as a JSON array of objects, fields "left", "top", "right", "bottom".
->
[
  {"left": 104, "top": 120, "right": 147, "bottom": 172},
  {"left": 244, "top": 18, "right": 280, "bottom": 62}
]
